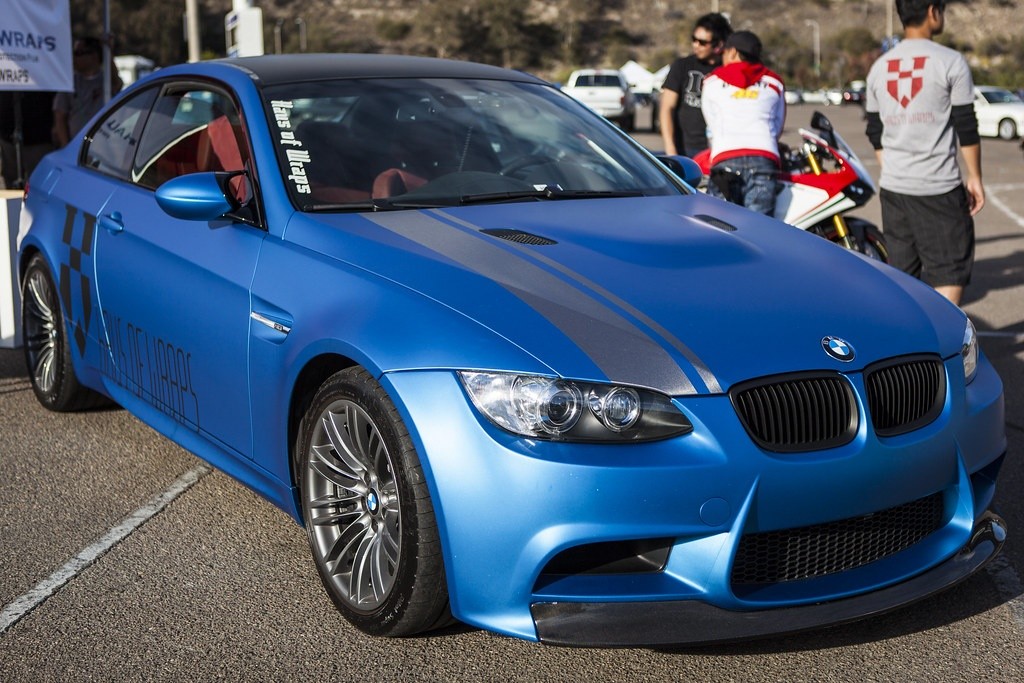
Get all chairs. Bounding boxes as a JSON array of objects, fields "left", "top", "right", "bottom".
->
[
  {"left": 197, "top": 125, "right": 251, "bottom": 172},
  {"left": 291, "top": 121, "right": 359, "bottom": 182}
]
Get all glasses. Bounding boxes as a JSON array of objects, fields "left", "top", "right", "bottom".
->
[{"left": 691, "top": 35, "right": 712, "bottom": 45}]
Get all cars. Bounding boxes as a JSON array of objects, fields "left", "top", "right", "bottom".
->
[
  {"left": 970, "top": 84, "right": 1024, "bottom": 143},
  {"left": 784, "top": 79, "right": 868, "bottom": 107},
  {"left": 16, "top": 53, "right": 1008, "bottom": 649}
]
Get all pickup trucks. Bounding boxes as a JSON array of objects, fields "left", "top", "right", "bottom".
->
[{"left": 559, "top": 68, "right": 637, "bottom": 134}]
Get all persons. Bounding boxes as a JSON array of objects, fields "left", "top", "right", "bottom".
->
[
  {"left": 661, "top": 12, "right": 735, "bottom": 160},
  {"left": 702, "top": 30, "right": 785, "bottom": 216},
  {"left": 866, "top": 0, "right": 986, "bottom": 306},
  {"left": 52, "top": 32, "right": 124, "bottom": 147}
]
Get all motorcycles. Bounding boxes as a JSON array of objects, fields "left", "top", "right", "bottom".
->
[{"left": 697, "top": 111, "right": 888, "bottom": 265}]
